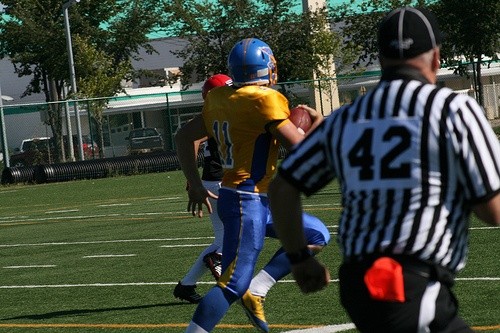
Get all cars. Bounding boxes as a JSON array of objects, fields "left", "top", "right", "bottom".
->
[
  {"left": 63, "top": 134, "right": 101, "bottom": 161},
  {"left": 16, "top": 136, "right": 52, "bottom": 152}
]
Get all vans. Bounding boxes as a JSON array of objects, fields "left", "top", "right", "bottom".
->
[{"left": 125, "top": 128, "right": 165, "bottom": 156}]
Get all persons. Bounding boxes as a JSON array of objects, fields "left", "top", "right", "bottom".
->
[
  {"left": 267, "top": 6, "right": 500, "bottom": 333},
  {"left": 173, "top": 37, "right": 330, "bottom": 333}
]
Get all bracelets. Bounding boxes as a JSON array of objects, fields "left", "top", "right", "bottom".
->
[{"left": 286, "top": 245, "right": 313, "bottom": 264}]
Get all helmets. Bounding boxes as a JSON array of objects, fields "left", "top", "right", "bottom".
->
[
  {"left": 202, "top": 74, "right": 233, "bottom": 99},
  {"left": 228, "top": 38, "right": 278, "bottom": 86}
]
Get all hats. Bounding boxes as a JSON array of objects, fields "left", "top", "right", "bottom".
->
[{"left": 377, "top": 7, "right": 449, "bottom": 60}]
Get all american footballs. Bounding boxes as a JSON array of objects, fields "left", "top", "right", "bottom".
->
[{"left": 290, "top": 107, "right": 313, "bottom": 137}]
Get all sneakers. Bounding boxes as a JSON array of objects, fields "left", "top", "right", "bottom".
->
[
  {"left": 203, "top": 249, "right": 222, "bottom": 282},
  {"left": 173, "top": 278, "right": 204, "bottom": 304},
  {"left": 237, "top": 288, "right": 268, "bottom": 333}
]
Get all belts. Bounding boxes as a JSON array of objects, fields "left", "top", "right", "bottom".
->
[{"left": 341, "top": 256, "right": 432, "bottom": 278}]
